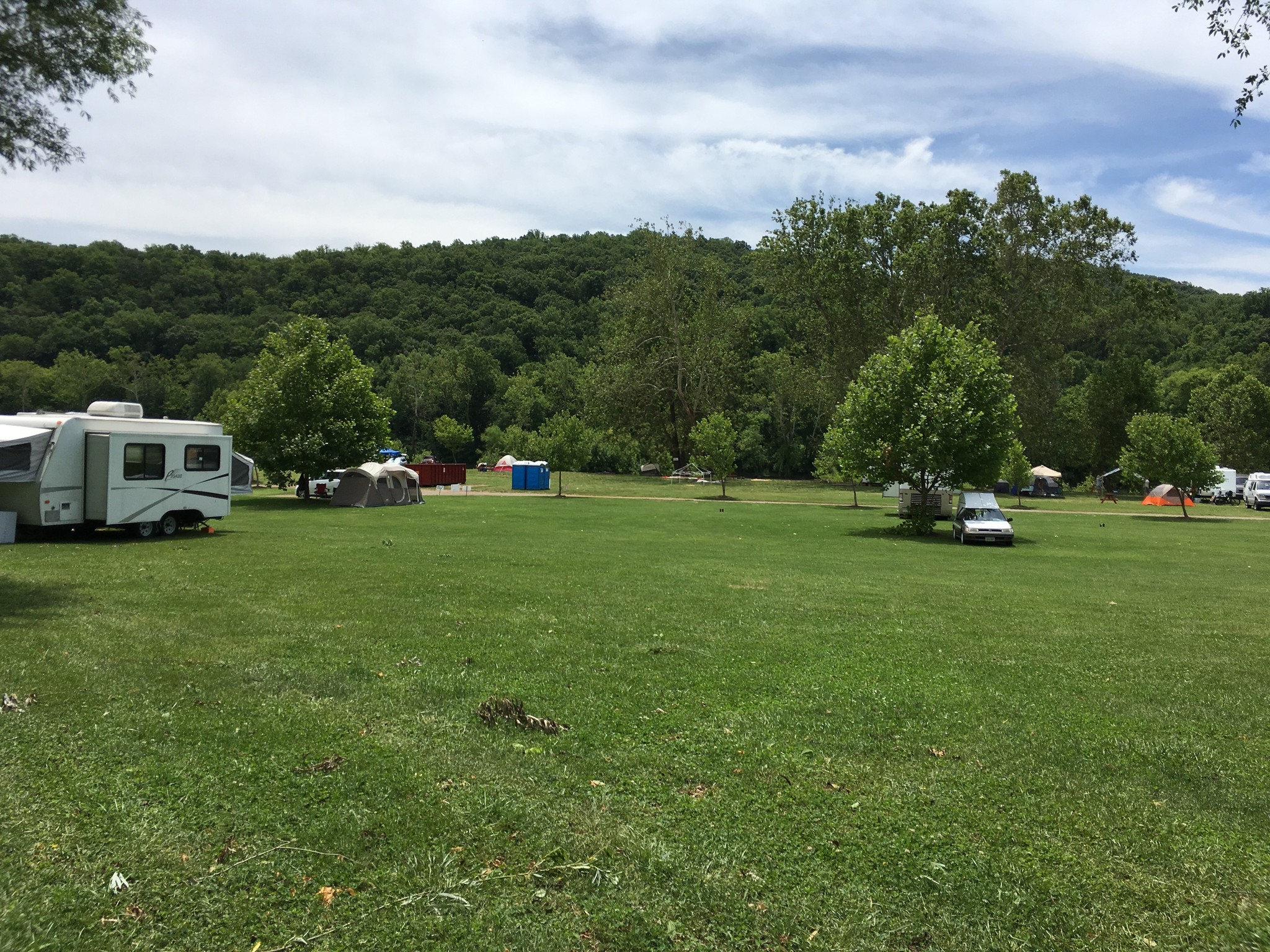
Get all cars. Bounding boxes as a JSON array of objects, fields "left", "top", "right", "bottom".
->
[
  {"left": 294, "top": 469, "right": 348, "bottom": 498},
  {"left": 952, "top": 507, "right": 1015, "bottom": 544}
]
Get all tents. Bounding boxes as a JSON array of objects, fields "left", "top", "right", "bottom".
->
[
  {"left": 494, "top": 455, "right": 516, "bottom": 471},
  {"left": 1141, "top": 484, "right": 1196, "bottom": 507},
  {"left": 327, "top": 449, "right": 425, "bottom": 509},
  {"left": 1022, "top": 465, "right": 1065, "bottom": 498}
]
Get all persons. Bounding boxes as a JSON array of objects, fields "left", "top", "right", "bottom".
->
[
  {"left": 1144, "top": 480, "right": 1149, "bottom": 496},
  {"left": 1096, "top": 475, "right": 1101, "bottom": 496}
]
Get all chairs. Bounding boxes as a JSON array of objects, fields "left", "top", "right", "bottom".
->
[{"left": 314, "top": 483, "right": 330, "bottom": 500}]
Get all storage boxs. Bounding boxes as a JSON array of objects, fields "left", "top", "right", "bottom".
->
[{"left": 403, "top": 464, "right": 466, "bottom": 487}]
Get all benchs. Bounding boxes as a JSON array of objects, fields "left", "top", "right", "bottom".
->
[
  {"left": 1089, "top": 488, "right": 1102, "bottom": 493},
  {"left": 1100, "top": 499, "right": 1118, "bottom": 504}
]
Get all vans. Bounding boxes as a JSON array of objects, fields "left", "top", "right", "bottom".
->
[{"left": 1235, "top": 472, "right": 1270, "bottom": 510}]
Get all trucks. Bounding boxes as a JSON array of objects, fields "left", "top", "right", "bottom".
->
[{"left": 0, "top": 401, "right": 233, "bottom": 538}]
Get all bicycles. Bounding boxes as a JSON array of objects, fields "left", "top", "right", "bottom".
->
[{"left": 1214, "top": 491, "right": 1241, "bottom": 506}]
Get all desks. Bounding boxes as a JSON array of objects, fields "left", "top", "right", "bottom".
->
[
  {"left": 1101, "top": 493, "right": 1117, "bottom": 504},
  {"left": 1091, "top": 484, "right": 1102, "bottom": 494}
]
